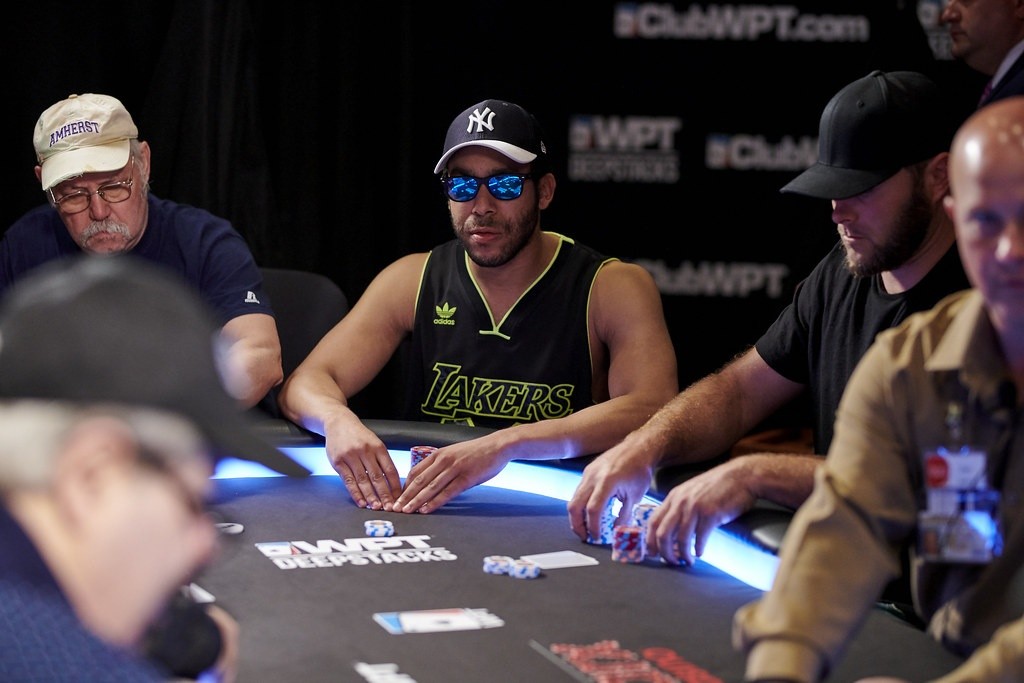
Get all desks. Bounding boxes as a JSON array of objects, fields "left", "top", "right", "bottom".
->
[{"left": 190, "top": 416, "right": 979, "bottom": 683}]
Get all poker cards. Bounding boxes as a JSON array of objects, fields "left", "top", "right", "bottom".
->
[
  {"left": 372, "top": 607, "right": 508, "bottom": 636},
  {"left": 521, "top": 548, "right": 600, "bottom": 570}
]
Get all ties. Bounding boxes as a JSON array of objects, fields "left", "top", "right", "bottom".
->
[{"left": 979, "top": 80, "right": 993, "bottom": 104}]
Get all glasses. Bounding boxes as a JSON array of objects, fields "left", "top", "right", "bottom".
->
[
  {"left": 137, "top": 444, "right": 242, "bottom": 564},
  {"left": 49, "top": 147, "right": 135, "bottom": 215},
  {"left": 441, "top": 169, "right": 535, "bottom": 202}
]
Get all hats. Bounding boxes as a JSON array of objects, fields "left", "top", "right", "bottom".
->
[
  {"left": 434, "top": 99, "right": 547, "bottom": 175},
  {"left": 33, "top": 92, "right": 138, "bottom": 190},
  {"left": 780, "top": 71, "right": 956, "bottom": 200},
  {"left": 1, "top": 259, "right": 314, "bottom": 476}
]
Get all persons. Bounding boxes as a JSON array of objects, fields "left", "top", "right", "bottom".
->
[
  {"left": 0, "top": 92, "right": 284, "bottom": 414},
  {"left": 566, "top": 69, "right": 974, "bottom": 629},
  {"left": 923, "top": 529, "right": 938, "bottom": 553},
  {"left": 278, "top": 99, "right": 679, "bottom": 514},
  {"left": 1, "top": 261, "right": 312, "bottom": 683},
  {"left": 731, "top": 101, "right": 1024, "bottom": 683},
  {"left": 941, "top": 0, "right": 1024, "bottom": 113}
]
